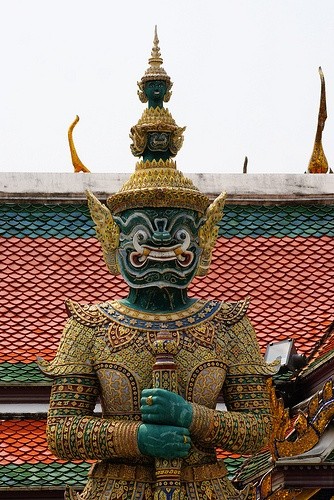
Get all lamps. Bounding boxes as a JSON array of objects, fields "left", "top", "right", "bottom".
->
[{"left": 263, "top": 338, "right": 306, "bottom": 374}]
